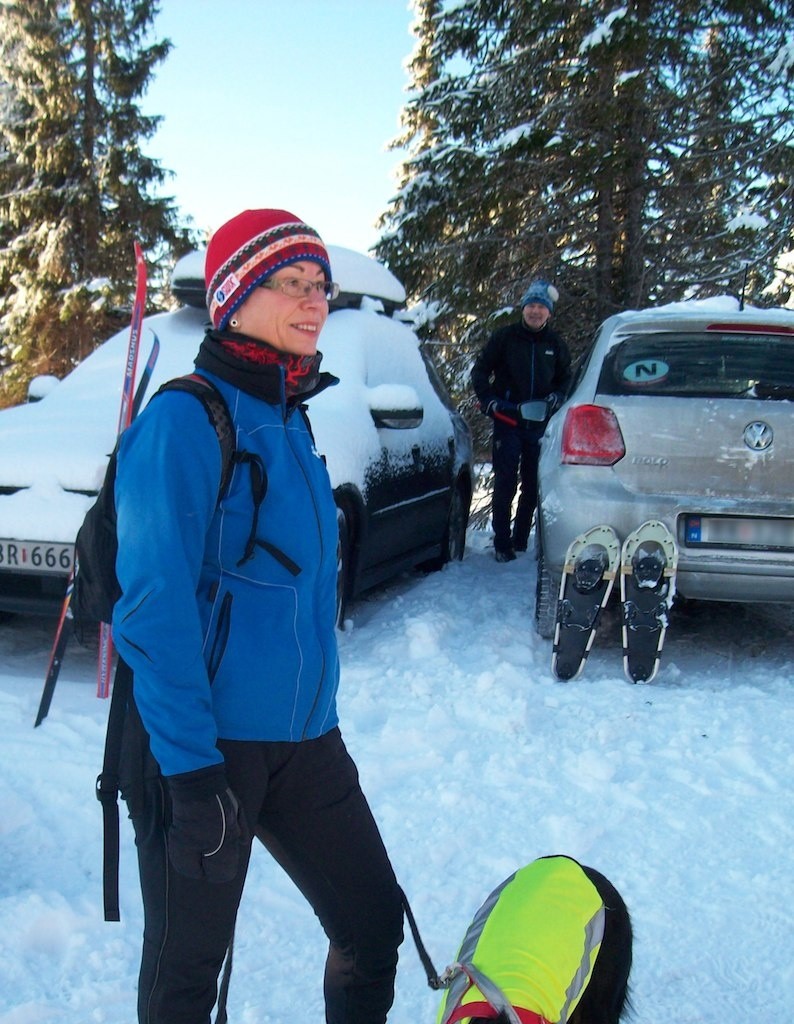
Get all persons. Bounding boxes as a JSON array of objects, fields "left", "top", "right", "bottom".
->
[
  {"left": 470, "top": 281, "right": 571, "bottom": 563},
  {"left": 108, "top": 210, "right": 408, "bottom": 1024}
]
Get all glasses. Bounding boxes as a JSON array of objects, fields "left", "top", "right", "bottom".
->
[{"left": 256, "top": 276, "right": 342, "bottom": 304}]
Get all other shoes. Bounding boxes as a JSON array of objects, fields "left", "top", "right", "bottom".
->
[{"left": 493, "top": 537, "right": 528, "bottom": 564}]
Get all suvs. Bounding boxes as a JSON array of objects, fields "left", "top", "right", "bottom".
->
[
  {"left": 0, "top": 247, "right": 474, "bottom": 628},
  {"left": 516, "top": 310, "right": 794, "bottom": 639}
]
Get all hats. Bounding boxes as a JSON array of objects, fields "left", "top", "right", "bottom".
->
[
  {"left": 207, "top": 207, "right": 331, "bottom": 332},
  {"left": 522, "top": 280, "right": 558, "bottom": 314}
]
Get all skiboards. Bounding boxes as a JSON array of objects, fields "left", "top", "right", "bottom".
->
[{"left": 35, "top": 239, "right": 160, "bottom": 727}]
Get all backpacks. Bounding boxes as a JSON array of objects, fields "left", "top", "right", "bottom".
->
[{"left": 69, "top": 377, "right": 239, "bottom": 651}]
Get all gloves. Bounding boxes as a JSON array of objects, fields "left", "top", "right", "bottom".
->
[
  {"left": 486, "top": 401, "right": 517, "bottom": 426},
  {"left": 169, "top": 788, "right": 250, "bottom": 884}
]
